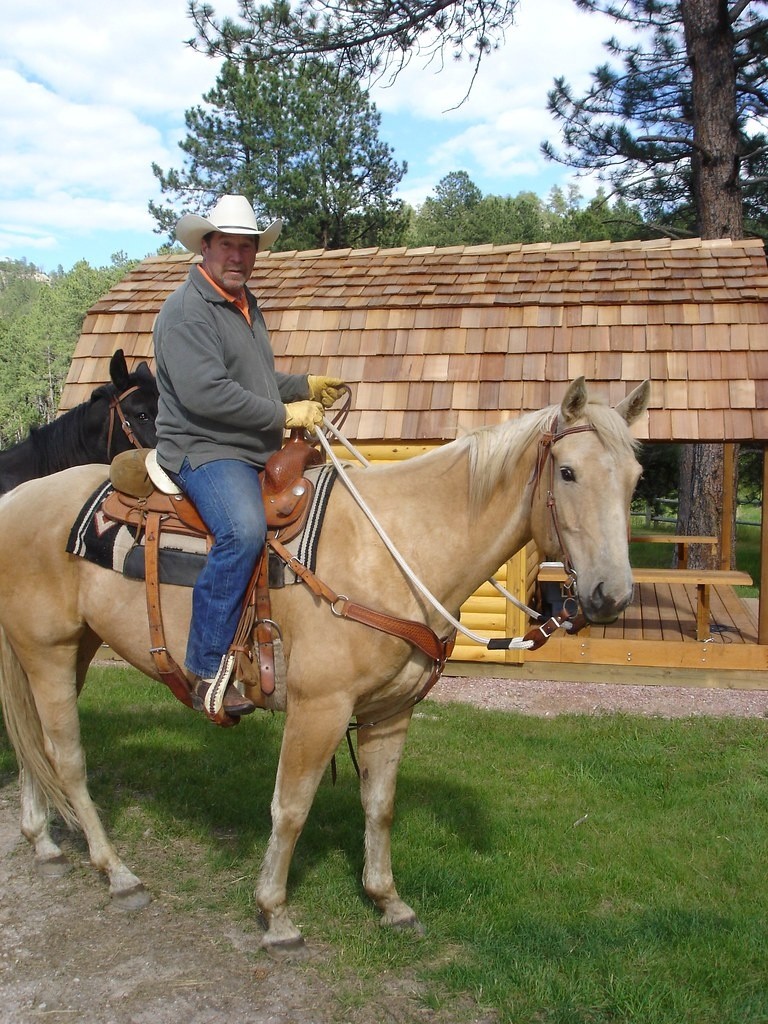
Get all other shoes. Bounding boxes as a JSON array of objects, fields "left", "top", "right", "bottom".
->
[{"left": 191, "top": 674, "right": 256, "bottom": 716}]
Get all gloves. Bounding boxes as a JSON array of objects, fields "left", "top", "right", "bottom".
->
[
  {"left": 284, "top": 400, "right": 325, "bottom": 438},
  {"left": 307, "top": 374, "right": 347, "bottom": 407}
]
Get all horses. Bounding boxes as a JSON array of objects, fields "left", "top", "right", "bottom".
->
[
  {"left": 2, "top": 348, "right": 159, "bottom": 701},
  {"left": 1, "top": 376, "right": 654, "bottom": 950}
]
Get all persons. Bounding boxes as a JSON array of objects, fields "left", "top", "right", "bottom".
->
[{"left": 152, "top": 195, "right": 347, "bottom": 715}]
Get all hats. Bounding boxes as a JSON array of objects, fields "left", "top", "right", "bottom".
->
[{"left": 175, "top": 194, "right": 282, "bottom": 255}]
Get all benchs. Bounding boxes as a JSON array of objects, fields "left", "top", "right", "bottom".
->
[
  {"left": 536, "top": 565, "right": 753, "bottom": 643},
  {"left": 632, "top": 536, "right": 719, "bottom": 569}
]
